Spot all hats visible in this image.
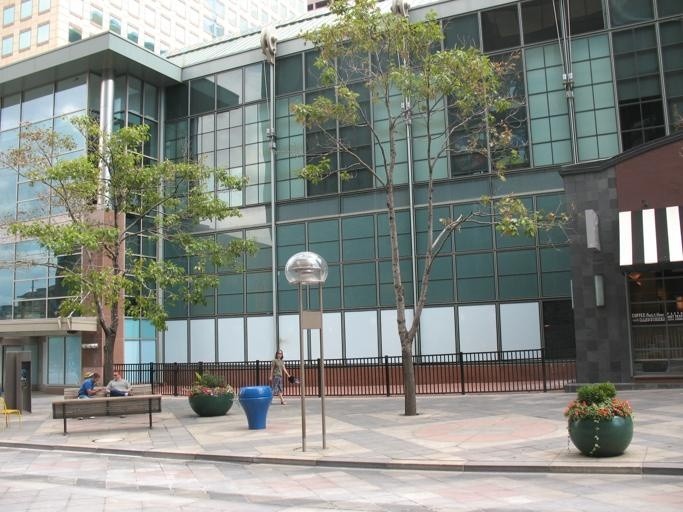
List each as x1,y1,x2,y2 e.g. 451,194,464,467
84,372,94,378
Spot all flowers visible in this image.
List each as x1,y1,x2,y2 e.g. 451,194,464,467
563,381,633,454
190,375,234,397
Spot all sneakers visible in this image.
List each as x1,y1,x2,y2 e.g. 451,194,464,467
128,391,135,396
281,400,288,406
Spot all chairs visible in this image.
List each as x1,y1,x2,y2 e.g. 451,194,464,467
0,398,20,428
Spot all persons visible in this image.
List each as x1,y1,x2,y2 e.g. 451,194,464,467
104,370,131,419
75,373,102,420
267,349,291,405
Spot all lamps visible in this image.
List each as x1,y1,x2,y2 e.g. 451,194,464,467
594,274,605,307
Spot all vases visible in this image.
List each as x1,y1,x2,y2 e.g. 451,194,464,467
643,360,668,373
569,414,632,457
189,396,234,417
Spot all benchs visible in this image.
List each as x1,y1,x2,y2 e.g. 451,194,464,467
64,384,152,400
53,394,162,433
